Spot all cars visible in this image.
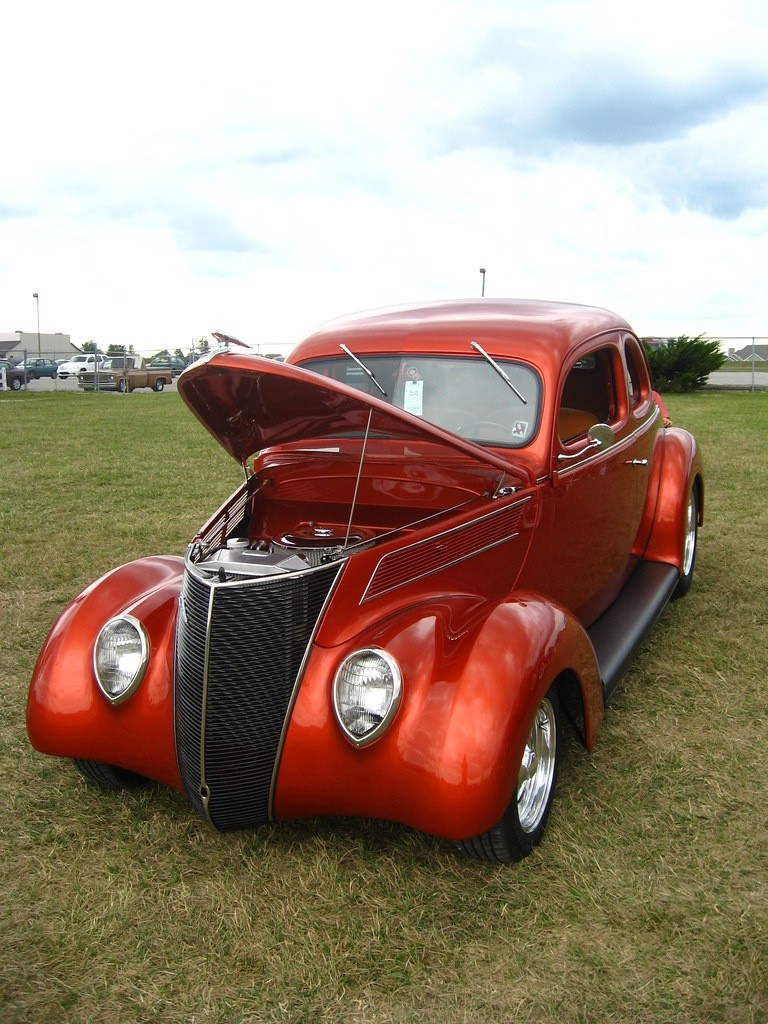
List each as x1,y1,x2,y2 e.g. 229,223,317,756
26,298,705,864
0,354,189,392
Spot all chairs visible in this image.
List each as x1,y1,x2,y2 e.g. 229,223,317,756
416,408,478,439
487,407,601,442
